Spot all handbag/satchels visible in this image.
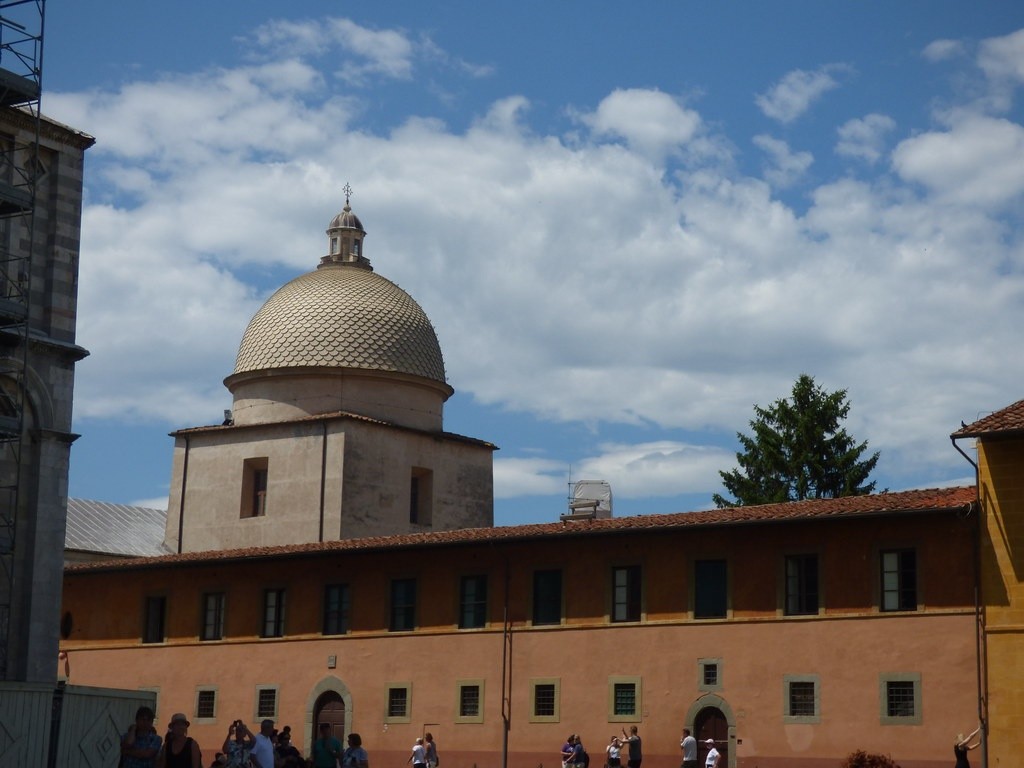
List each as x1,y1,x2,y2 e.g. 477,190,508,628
583,752,589,768
435,756,440,767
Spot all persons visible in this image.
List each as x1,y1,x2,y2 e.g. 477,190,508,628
160,713,203,768
560,734,585,768
953,725,981,768
222,719,255,768
424,732,438,768
120,706,162,768
408,737,428,768
211,753,225,768
338,733,368,768
606,726,642,768
704,739,721,768
311,723,344,768
251,719,307,768
679,728,697,768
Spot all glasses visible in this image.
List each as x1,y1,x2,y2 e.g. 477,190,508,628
282,737,290,741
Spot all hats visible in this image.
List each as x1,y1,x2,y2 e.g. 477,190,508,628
168,713,191,729
704,739,714,744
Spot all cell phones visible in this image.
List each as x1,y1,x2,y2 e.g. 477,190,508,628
233,721,238,727
